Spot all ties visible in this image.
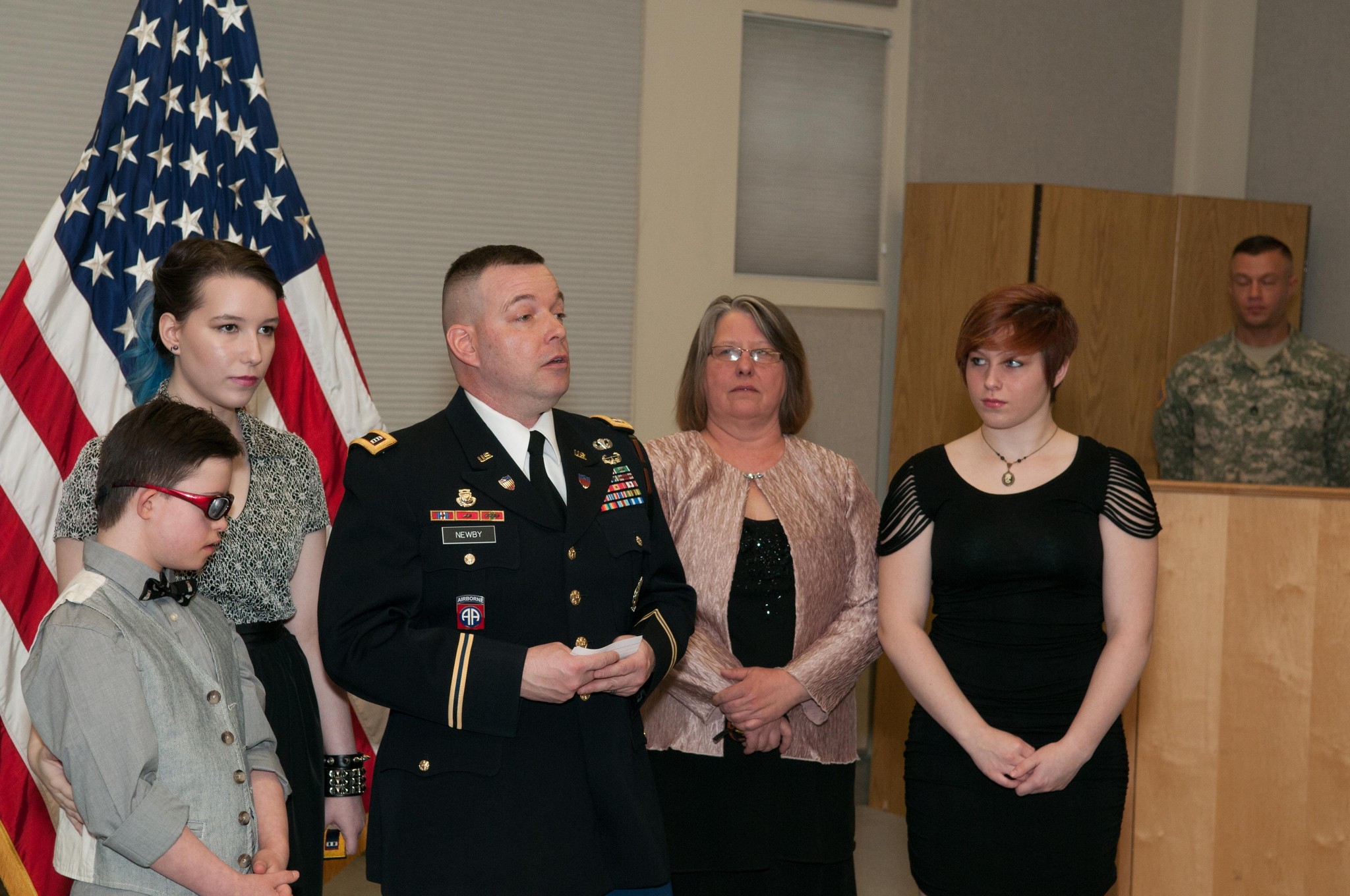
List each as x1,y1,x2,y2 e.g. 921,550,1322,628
527,428,567,520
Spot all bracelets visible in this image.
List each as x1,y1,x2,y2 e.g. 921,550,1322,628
324,752,371,798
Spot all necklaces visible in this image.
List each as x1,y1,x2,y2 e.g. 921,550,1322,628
981,425,1058,486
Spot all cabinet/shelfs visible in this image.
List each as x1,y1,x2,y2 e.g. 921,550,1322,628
869,180,1311,813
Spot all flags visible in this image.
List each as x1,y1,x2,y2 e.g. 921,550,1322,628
0,0,390,896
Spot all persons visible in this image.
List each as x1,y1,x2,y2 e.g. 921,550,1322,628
26,238,369,896
20,397,300,896
875,282,1162,896
1150,235,1350,489
318,245,699,896
627,295,884,896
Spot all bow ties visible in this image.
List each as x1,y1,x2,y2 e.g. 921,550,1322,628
138,572,197,607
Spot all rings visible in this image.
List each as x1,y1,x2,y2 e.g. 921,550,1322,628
611,690,617,694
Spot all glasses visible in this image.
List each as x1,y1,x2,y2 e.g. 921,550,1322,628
112,480,234,521
708,345,784,364
